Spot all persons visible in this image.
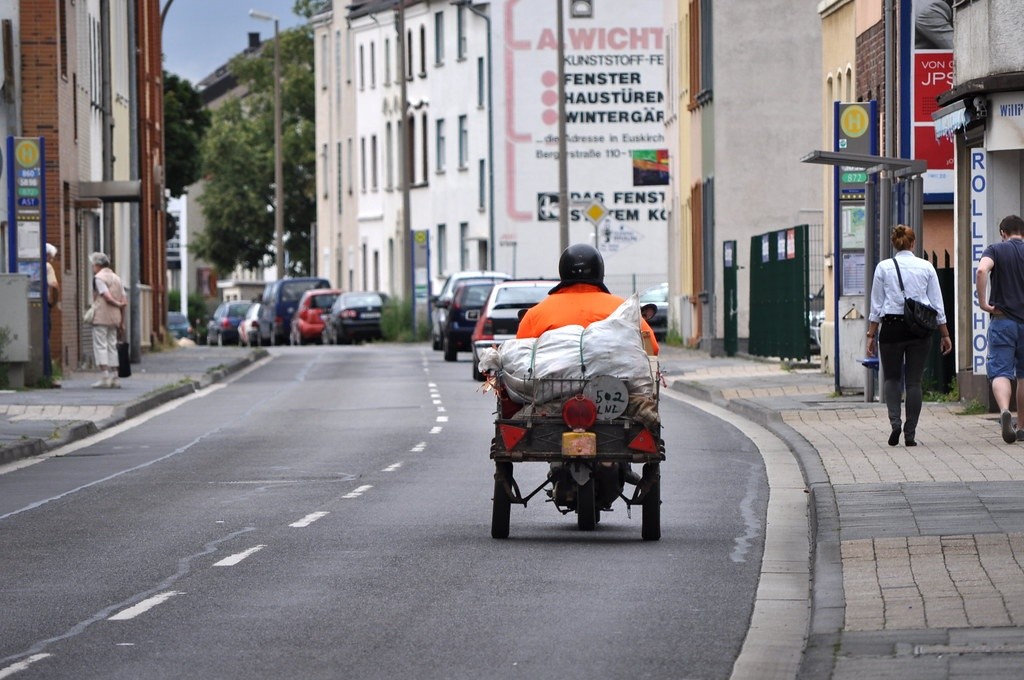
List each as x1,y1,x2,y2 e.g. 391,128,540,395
866,225,952,446
45,243,61,388
84,252,128,389
976,215,1024,444
516,243,660,501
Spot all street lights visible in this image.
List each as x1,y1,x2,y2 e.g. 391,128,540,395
249,9,284,282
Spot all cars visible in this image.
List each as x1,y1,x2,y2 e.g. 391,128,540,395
204,300,255,347
638,282,669,334
237,303,262,348
166,311,197,342
427,271,513,350
434,280,505,362
287,288,344,347
323,291,389,345
466,279,563,382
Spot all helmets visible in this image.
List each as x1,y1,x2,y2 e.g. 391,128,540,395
558,243,605,282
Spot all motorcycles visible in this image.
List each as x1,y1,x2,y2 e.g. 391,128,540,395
489,304,666,542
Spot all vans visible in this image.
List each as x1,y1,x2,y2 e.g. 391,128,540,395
255,275,331,347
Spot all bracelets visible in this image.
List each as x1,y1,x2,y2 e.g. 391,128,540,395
941,335,949,337
867,331,874,337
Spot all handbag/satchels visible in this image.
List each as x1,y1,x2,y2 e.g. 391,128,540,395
116,331,131,377
904,298,938,338
83,307,95,323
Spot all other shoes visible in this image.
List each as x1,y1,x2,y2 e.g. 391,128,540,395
626,463,640,485
1016,429,1024,441
91,381,112,388
112,381,121,388
52,380,61,388
905,441,918,446
888,425,902,446
998,409,1015,444
